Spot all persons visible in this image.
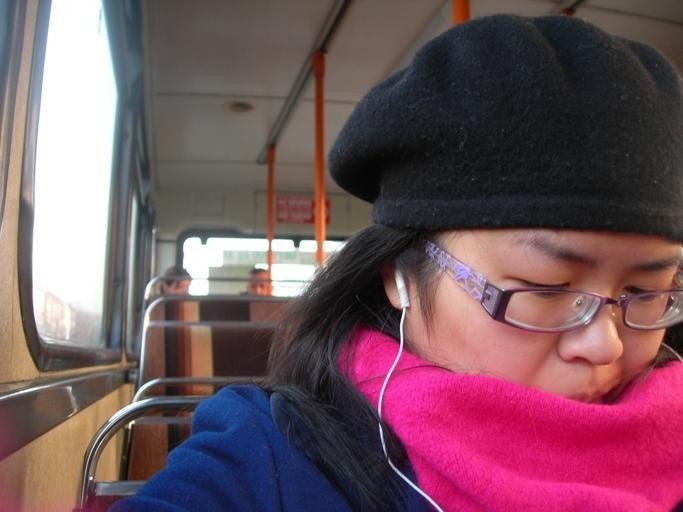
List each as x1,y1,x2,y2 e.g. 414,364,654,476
106,14,683,510
237,268,273,296
156,264,192,295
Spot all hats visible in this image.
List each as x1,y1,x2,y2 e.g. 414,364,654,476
331,13,682,236
163,266,192,280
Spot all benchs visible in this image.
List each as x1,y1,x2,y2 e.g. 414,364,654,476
78,277,302,511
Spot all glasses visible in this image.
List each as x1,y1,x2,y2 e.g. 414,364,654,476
417,240,683,332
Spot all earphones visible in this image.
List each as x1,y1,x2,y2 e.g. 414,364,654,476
391,261,410,309
